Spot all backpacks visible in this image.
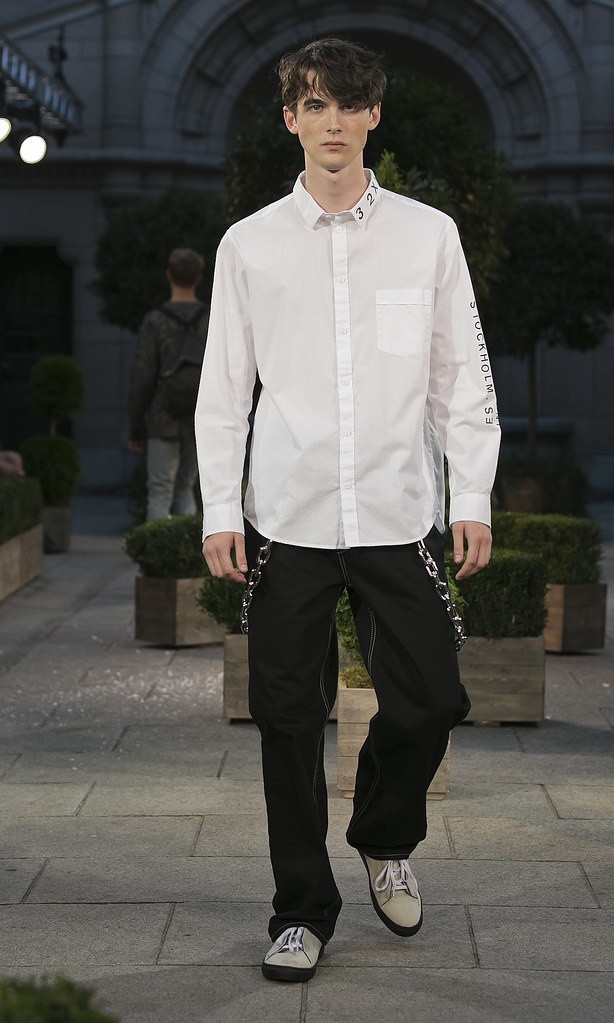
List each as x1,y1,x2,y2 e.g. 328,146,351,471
161,308,208,419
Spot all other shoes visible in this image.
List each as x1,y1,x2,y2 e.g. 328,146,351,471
261,923,326,981
357,851,424,938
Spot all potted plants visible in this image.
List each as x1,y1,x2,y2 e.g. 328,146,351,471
496,459,551,512
332,567,469,801
23,434,82,554
197,570,251,723
509,513,607,655
121,515,228,646
0,475,47,600
444,548,549,727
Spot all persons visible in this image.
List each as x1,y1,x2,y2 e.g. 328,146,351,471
191,35,505,983
126,250,213,525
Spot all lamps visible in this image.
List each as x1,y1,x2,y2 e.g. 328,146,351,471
11,127,48,163
